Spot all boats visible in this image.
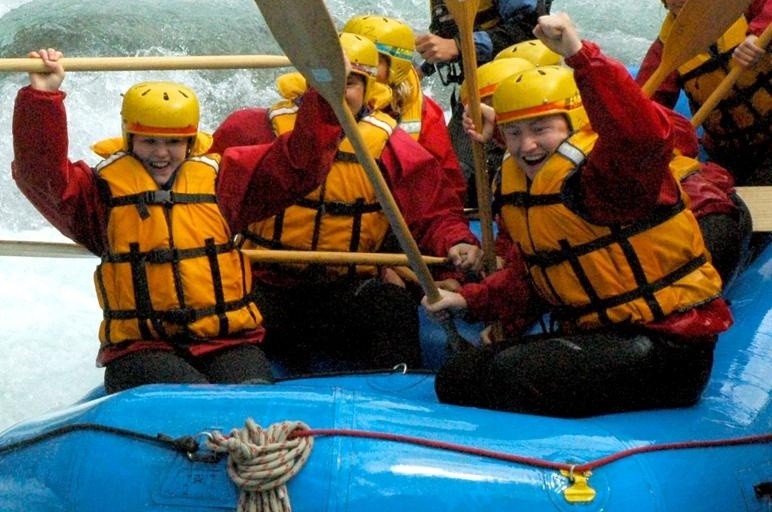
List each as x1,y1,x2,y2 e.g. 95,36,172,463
1,38,772,511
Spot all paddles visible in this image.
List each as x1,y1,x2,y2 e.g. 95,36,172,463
258,1,454,344
639,0,751,95
0,239,445,268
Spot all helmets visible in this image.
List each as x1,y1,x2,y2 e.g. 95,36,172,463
462,38,590,132
338,30,377,110
122,82,199,160
345,16,415,90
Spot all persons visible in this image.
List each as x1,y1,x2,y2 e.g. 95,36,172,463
210,1,752,415
10,47,350,394
634,1,772,184
